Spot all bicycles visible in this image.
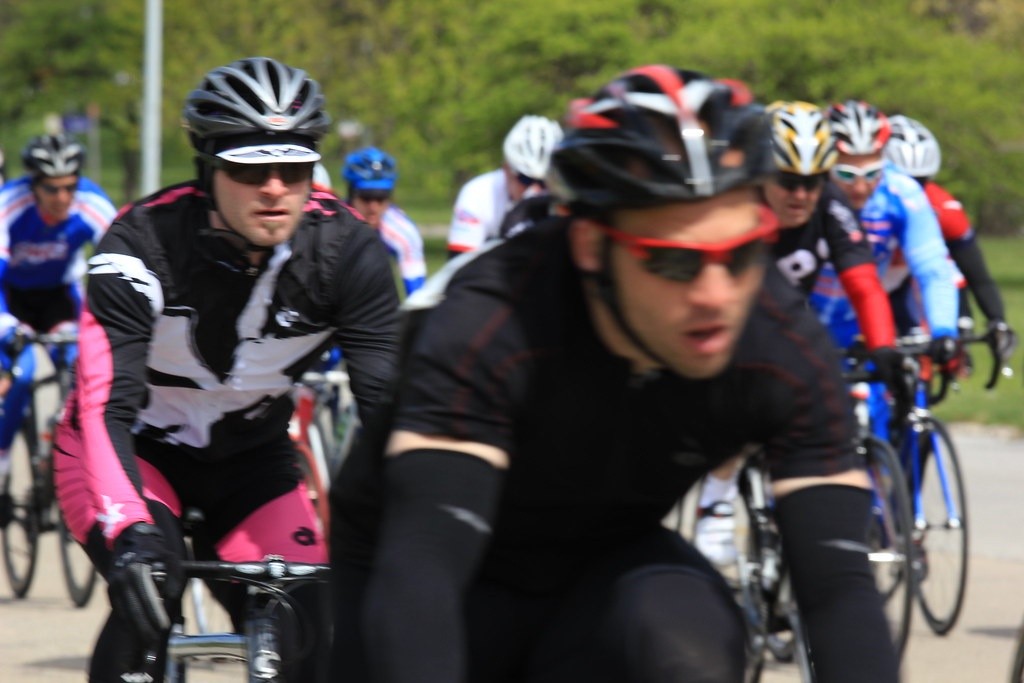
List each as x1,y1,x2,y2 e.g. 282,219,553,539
0,309,1013,683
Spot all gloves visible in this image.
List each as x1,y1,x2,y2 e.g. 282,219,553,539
928,336,956,363
869,347,910,419
108,521,180,637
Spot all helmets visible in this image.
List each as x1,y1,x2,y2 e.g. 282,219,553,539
823,100,892,154
544,66,774,207
879,115,940,176
23,133,84,177
503,115,563,179
342,148,396,189
183,57,332,164
764,101,840,174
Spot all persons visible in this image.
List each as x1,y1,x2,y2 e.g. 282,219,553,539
0,57,1014,683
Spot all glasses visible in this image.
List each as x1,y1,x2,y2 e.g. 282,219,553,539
588,208,777,282
40,182,78,193
772,170,818,191
830,164,882,182
218,163,314,185
358,189,389,200
515,172,545,190
